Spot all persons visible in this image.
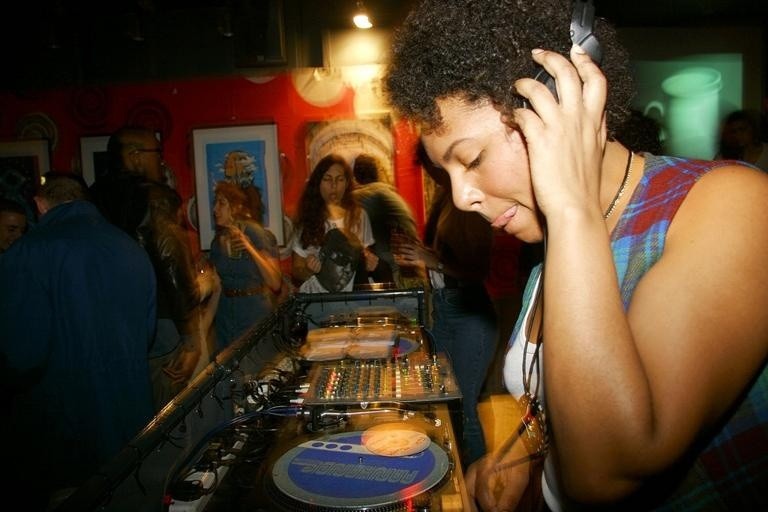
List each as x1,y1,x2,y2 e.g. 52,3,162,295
712,107,768,172
614,107,665,154
378,0,766,511
1,121,502,512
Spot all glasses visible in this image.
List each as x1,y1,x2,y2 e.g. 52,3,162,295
325,247,356,269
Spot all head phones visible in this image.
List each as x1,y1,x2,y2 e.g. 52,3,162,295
523,0,608,109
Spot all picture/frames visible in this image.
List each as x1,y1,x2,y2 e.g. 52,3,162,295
6,139,49,227
77,133,164,188
191,120,285,250
227,0,288,68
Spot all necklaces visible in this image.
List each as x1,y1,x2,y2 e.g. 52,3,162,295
514,150,636,463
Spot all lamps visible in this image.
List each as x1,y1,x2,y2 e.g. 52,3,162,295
352,0,377,29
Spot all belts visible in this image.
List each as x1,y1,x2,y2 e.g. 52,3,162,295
224,287,271,295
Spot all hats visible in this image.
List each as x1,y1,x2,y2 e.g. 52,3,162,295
322,230,365,270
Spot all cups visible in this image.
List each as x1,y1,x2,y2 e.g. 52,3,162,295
389,222,410,254
224,221,240,259
643,66,723,168
